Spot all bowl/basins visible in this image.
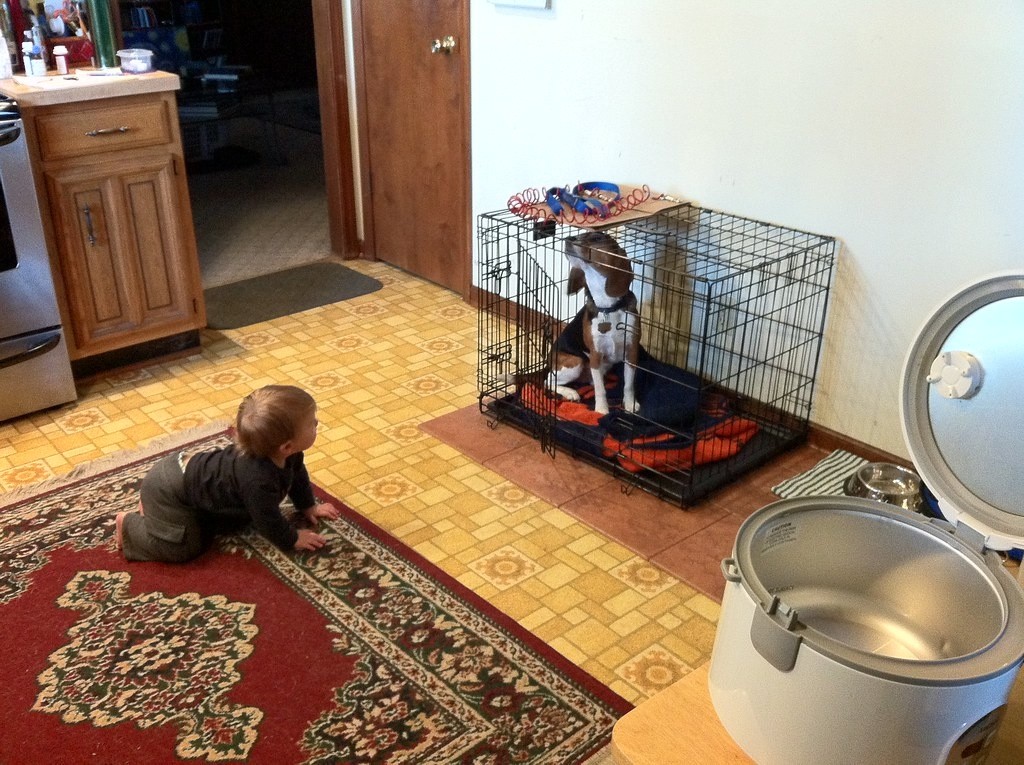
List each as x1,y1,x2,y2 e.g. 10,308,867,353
844,461,922,514
115,48,153,73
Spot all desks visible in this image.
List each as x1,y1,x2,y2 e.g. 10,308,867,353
611,555,1024,765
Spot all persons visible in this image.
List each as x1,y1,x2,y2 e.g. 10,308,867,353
115,385,341,562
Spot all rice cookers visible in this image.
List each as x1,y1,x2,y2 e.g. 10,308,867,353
707,260,1024,765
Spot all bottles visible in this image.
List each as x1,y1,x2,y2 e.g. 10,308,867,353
27,47,48,77
85,0,118,69
22,30,34,76
33,25,46,62
53,46,70,75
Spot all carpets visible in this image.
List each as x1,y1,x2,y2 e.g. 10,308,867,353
770,448,872,500
0,420,638,764
201,259,384,332
415,391,869,608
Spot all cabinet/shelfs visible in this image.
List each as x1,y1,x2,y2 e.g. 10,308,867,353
0,64,208,387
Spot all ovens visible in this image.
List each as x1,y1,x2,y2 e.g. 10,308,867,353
0,113,78,427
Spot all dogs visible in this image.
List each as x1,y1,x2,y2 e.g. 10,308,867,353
494,231,642,414
